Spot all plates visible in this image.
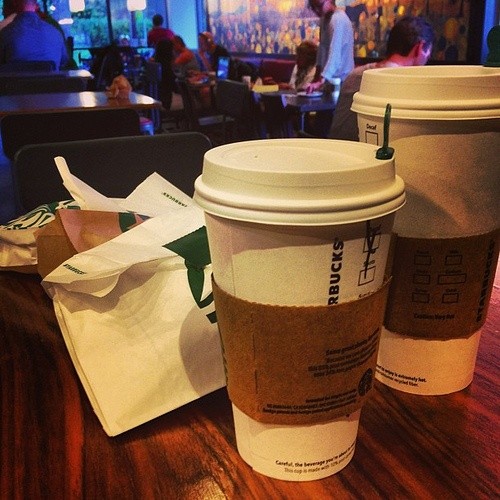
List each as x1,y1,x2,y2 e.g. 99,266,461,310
298,92,323,97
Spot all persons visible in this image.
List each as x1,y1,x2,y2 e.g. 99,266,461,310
114,13,234,134
264,41,317,91
0,0,69,71
328,16,435,141
306,0,354,128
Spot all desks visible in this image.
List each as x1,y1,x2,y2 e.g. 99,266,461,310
0,250,500,500
0,69,93,91
0,92,163,157
259,89,339,131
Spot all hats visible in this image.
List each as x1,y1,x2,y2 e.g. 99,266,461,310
199,30,214,43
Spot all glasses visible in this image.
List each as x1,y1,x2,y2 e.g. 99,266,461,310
307,0,326,11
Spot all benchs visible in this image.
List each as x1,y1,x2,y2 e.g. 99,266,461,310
230,56,296,82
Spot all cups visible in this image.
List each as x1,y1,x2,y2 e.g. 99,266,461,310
350,64,500,398
192,138,408,482
242,75,251,90
329,77,341,96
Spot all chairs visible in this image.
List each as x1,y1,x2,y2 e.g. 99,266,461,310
13,131,212,221
0,61,69,90
96,54,293,141
1,109,140,159
